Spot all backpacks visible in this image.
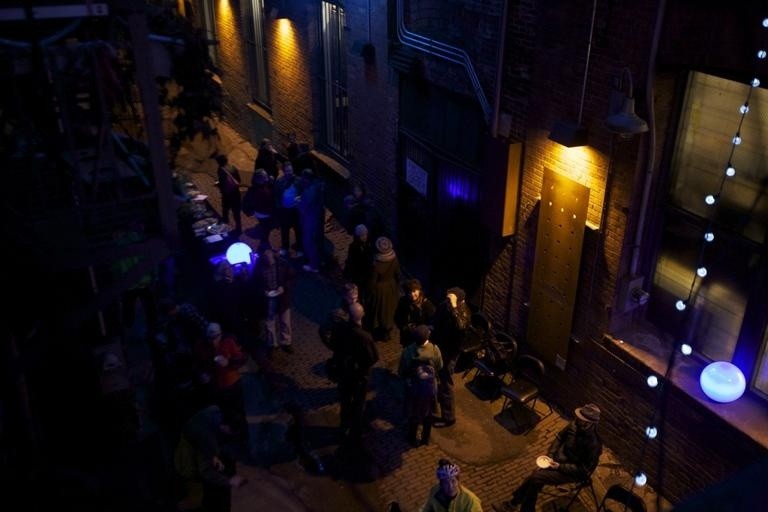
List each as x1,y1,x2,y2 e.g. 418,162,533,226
414,366,437,398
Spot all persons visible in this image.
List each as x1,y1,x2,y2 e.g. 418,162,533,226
490,403,603,512
0,3,346,512
345,183,470,448
419,458,482,511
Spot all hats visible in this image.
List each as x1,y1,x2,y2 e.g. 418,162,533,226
375,236,393,255
575,404,601,424
205,322,223,340
353,224,368,237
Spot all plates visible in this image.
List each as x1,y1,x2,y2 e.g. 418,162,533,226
535,455,552,470
268,290,280,298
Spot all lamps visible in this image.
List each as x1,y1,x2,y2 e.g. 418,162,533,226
548,116,593,148
270,6,287,19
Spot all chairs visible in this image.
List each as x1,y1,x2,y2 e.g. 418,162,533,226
454,313,553,435
557,459,647,511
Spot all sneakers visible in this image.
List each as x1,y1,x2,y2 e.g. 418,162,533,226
433,418,455,428
268,343,296,359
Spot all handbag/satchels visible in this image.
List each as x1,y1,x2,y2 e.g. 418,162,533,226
217,165,240,193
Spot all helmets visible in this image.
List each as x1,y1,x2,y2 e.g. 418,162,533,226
436,463,461,480
